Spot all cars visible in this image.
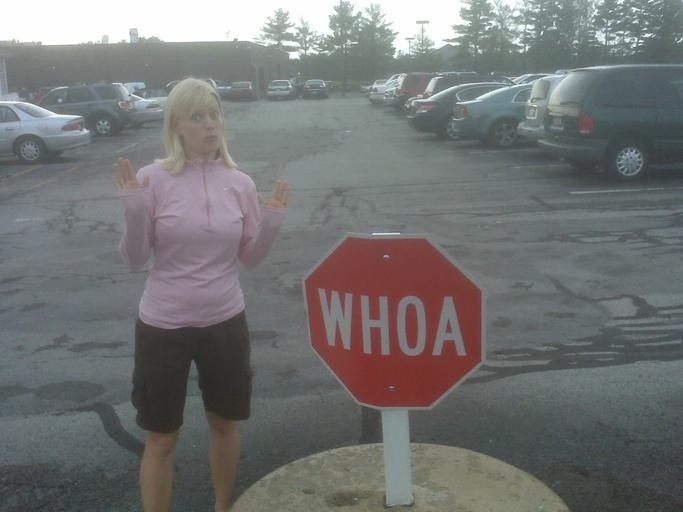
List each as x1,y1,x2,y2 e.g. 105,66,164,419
0,100,92,164
129,93,162,129
360,63,567,150
131,87,168,119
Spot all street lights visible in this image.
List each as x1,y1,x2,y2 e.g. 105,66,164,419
405,37,415,55
416,20,430,51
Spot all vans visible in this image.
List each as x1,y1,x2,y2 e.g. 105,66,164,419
536,63,682,181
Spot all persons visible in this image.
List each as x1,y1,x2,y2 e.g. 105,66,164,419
114,73,291,511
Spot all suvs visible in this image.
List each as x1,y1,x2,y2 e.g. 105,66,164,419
206,77,332,101
34,82,134,140
